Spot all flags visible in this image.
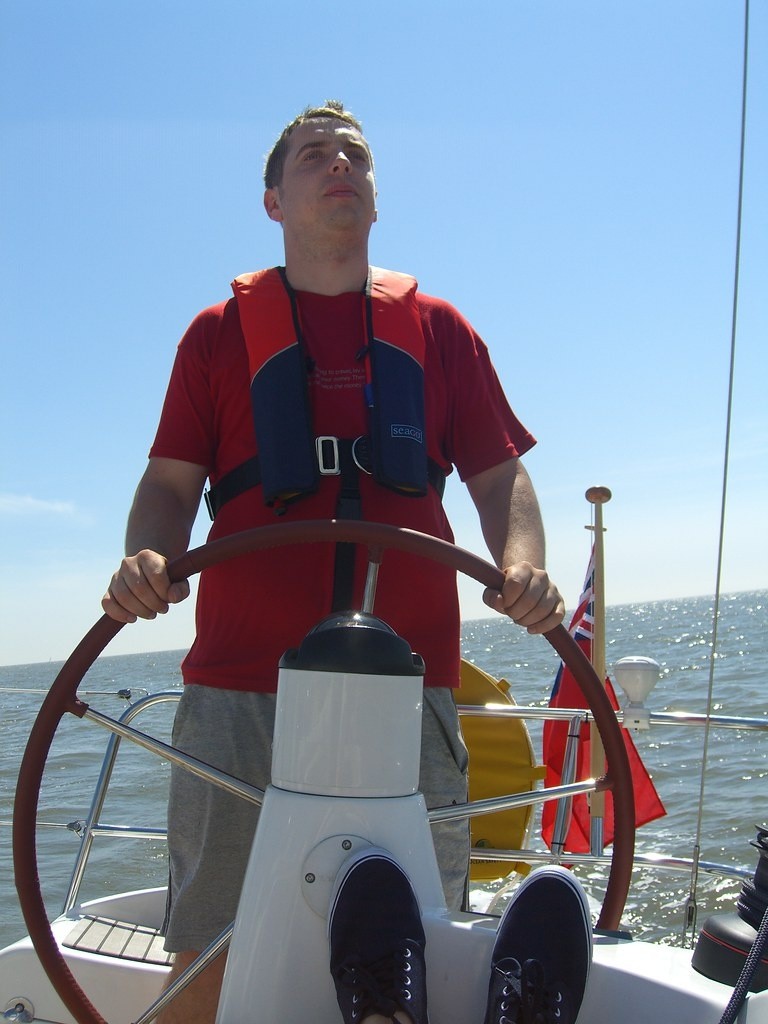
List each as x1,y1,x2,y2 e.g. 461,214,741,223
542,542,669,871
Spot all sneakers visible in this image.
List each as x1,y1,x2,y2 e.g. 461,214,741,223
328,845,431,1024
483,864,593,1024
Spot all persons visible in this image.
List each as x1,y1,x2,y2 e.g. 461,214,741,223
327,847,593,1024
100,103,565,1024
